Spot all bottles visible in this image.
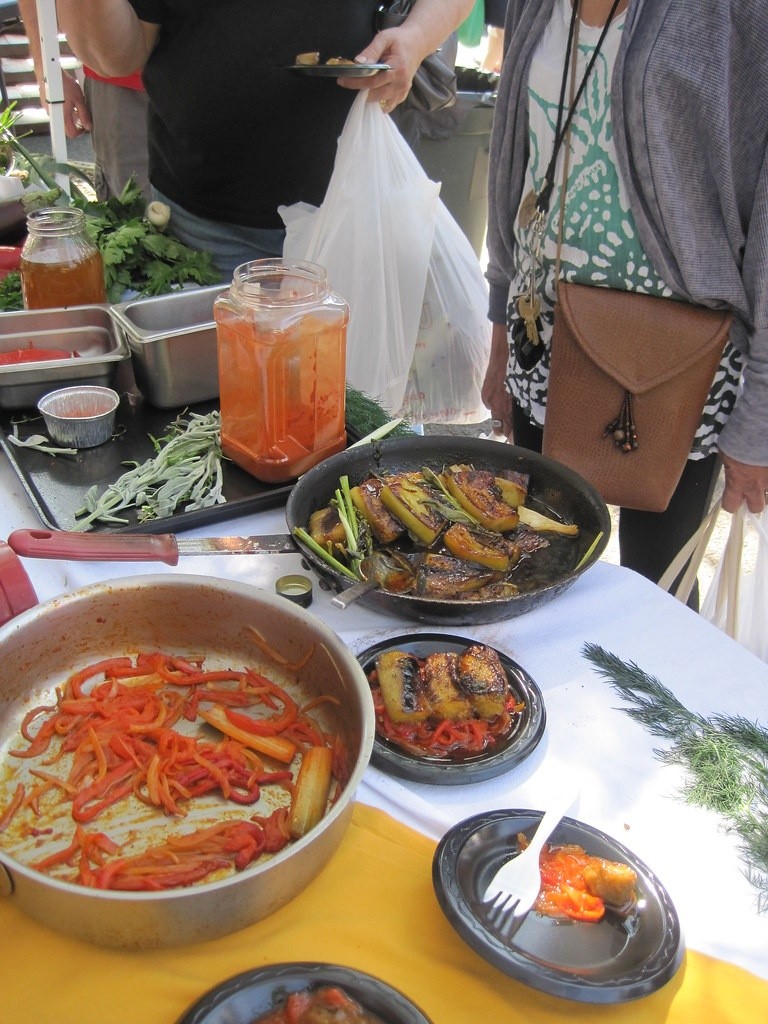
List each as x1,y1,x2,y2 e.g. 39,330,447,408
19,205,105,310
212,256,350,484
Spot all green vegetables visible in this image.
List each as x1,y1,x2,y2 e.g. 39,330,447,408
0,99,228,312
6,375,417,531
297,467,480,582
581,641,768,904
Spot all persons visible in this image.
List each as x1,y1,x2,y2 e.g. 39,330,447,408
481,0,768,613
18,0,153,222
56,0,474,298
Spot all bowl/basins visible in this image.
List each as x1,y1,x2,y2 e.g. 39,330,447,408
36,384,120,449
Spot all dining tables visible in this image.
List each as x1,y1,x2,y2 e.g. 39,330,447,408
0,283,768,1024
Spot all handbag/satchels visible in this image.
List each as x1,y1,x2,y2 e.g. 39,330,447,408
369,0,459,114
541,279,732,514
276,84,493,426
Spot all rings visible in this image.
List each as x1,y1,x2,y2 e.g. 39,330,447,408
381,99,387,109
765,492,768,494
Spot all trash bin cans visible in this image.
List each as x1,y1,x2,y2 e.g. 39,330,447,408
409,64,501,265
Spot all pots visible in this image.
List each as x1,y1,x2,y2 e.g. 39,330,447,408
0,572,376,953
6,435,612,629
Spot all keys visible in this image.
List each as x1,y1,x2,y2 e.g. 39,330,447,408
516,296,540,345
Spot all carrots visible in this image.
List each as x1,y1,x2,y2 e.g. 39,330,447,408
0,652,353,891
367,671,521,755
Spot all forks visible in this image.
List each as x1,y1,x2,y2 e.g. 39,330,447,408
481,783,576,919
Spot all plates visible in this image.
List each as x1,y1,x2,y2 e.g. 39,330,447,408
273,63,392,78
432,808,684,1005
356,633,548,786
175,962,432,1023
